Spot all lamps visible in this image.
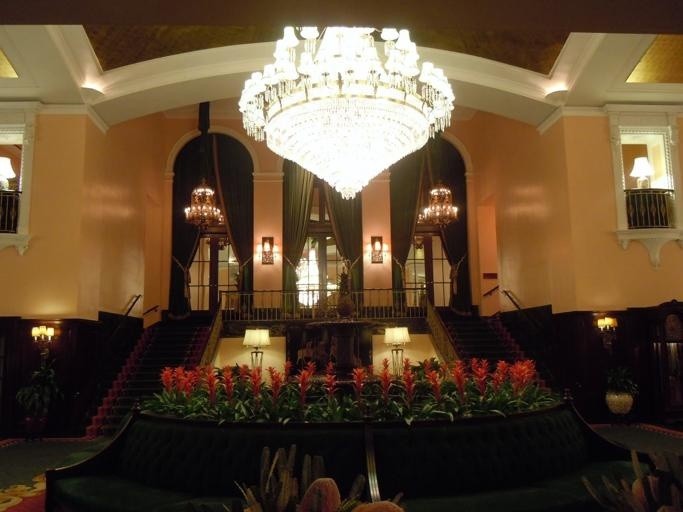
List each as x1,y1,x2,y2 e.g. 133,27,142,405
237,22,456,205
416,178,460,227
0,155,17,190
596,315,620,349
629,157,654,189
243,327,270,365
29,324,56,366
185,177,225,232
383,327,412,366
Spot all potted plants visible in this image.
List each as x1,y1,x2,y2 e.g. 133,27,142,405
14,359,66,442
602,366,640,415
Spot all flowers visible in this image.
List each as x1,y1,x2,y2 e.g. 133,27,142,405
147,358,553,422
